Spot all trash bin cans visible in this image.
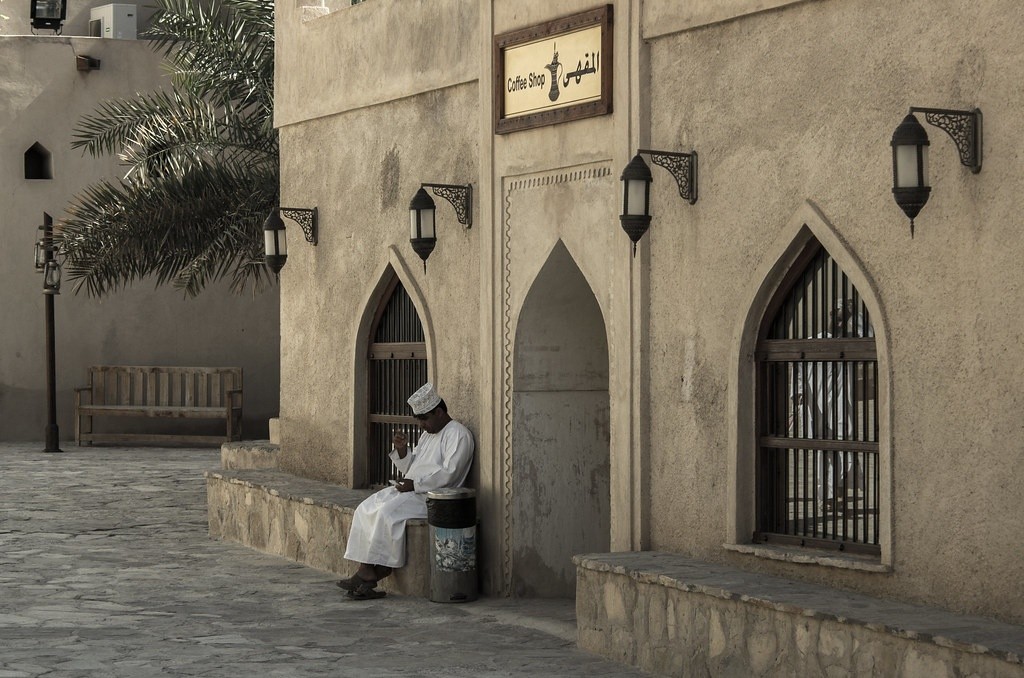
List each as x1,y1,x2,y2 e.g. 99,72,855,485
427,487,479,602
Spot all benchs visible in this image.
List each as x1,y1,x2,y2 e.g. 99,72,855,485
72,365,246,452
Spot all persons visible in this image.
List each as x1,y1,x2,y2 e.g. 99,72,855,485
791,298,862,511
336,383,474,599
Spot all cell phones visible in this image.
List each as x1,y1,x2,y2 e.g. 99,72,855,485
389,480,401,486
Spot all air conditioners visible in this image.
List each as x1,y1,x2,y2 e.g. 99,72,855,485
88,3,138,40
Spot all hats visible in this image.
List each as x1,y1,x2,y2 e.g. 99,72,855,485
407,383,441,415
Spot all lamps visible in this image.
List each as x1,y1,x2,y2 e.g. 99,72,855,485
620,149,698,257
889,107,984,241
263,206,319,286
29,0,67,35
407,182,474,274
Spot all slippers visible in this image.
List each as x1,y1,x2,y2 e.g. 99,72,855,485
345,584,386,599
336,573,377,590
